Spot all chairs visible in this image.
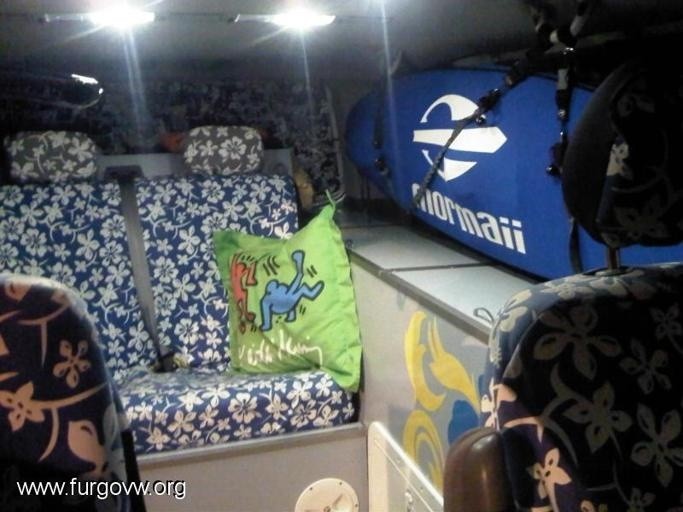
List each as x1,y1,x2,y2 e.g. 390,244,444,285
0,75,365,511
448,46,683,512
